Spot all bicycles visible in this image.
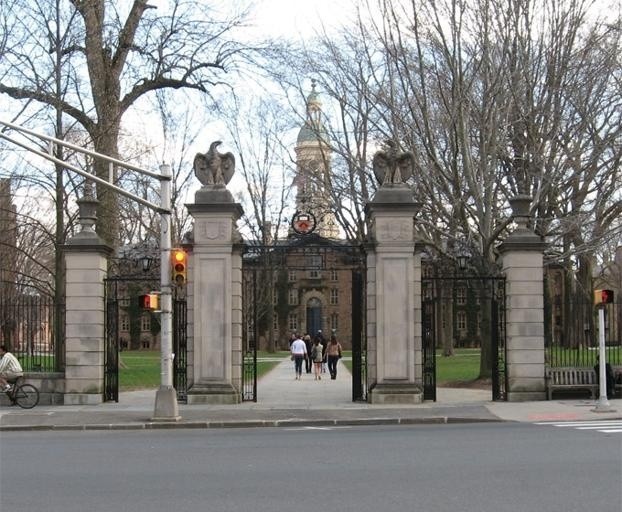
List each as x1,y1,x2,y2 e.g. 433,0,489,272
0,375,41,409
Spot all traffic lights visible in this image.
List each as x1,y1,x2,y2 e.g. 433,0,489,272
593,288,616,307
172,250,187,285
144,293,158,310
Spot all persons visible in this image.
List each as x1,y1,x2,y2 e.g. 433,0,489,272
594,355,616,396
288,328,343,380
0,345,24,394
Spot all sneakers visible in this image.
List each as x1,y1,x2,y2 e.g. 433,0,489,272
1,384,11,392
295,368,337,380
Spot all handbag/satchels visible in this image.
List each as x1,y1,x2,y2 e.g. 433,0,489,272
338,351,342,358
311,349,317,359
